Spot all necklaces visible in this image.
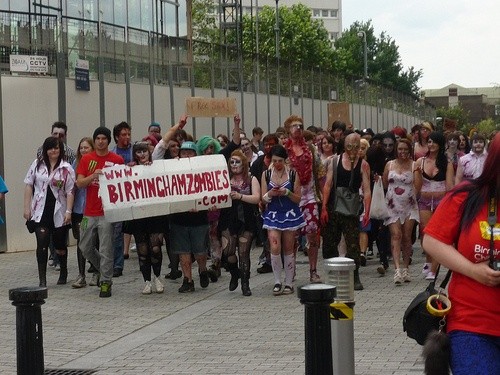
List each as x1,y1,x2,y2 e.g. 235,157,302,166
280,169,285,177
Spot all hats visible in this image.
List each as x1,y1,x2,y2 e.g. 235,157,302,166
361,128,374,137
93,126,111,145
180,141,196,152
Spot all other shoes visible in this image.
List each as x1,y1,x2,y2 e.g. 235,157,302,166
360,254,366,266
39,255,323,297
402,268,411,282
354,275,363,290
377,265,385,274
422,262,432,274
425,272,436,280
366,251,373,258
393,268,401,284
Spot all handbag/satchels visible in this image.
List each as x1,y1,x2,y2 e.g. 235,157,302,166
333,187,360,216
360,176,391,221
26,218,35,233
403,290,446,345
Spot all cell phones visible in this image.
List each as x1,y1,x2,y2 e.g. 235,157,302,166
273,186,280,189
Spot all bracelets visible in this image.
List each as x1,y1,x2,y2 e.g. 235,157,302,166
284,190,290,195
239,195,242,200
66,209,72,214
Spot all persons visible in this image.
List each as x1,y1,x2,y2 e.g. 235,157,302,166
422,131,500,375
0,177,8,203
24,117,487,296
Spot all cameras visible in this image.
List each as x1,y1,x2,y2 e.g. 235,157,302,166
489,259,500,271
